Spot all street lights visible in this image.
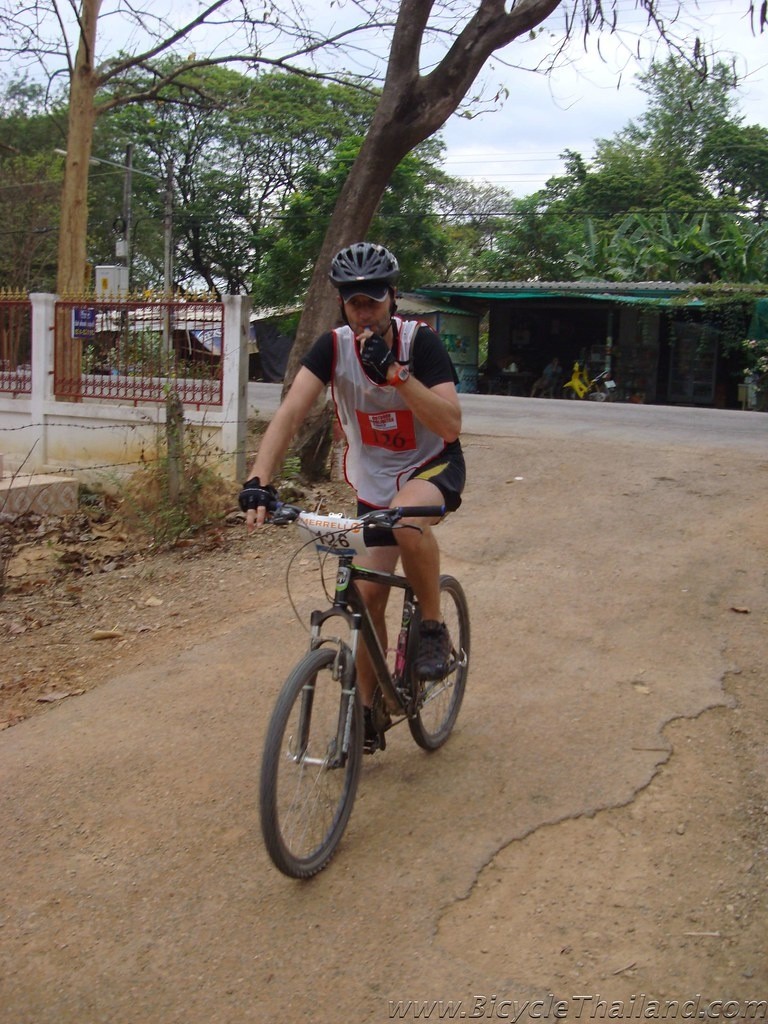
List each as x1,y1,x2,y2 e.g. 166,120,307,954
53,148,171,376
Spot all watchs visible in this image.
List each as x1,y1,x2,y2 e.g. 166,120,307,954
389,366,409,386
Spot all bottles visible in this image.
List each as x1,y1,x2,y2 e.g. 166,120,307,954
360,325,374,354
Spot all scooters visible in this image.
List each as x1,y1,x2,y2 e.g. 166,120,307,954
562,359,621,403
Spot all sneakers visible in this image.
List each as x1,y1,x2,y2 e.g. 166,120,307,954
413,620,449,680
363,705,392,747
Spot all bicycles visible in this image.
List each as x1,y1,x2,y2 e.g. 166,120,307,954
232,502,470,879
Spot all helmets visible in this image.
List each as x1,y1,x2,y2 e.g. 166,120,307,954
328,242,400,288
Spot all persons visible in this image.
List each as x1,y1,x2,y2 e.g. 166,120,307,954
529,356,563,397
237,241,467,751
